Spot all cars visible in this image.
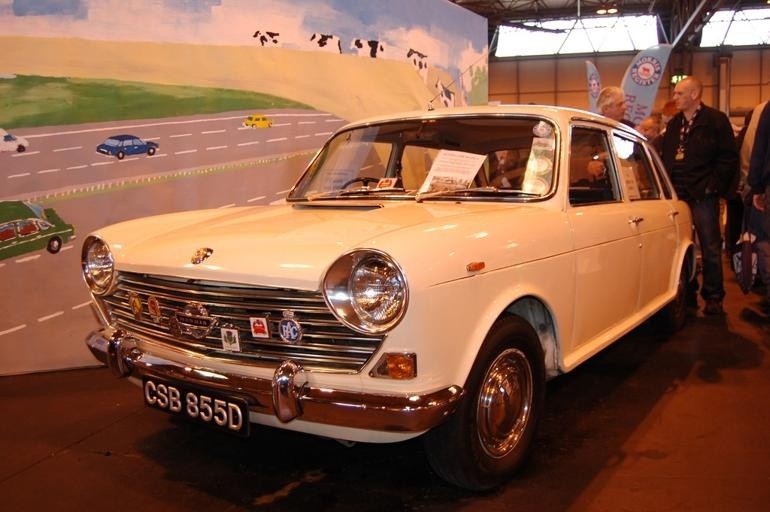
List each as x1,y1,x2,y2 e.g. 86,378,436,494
81,101,696,490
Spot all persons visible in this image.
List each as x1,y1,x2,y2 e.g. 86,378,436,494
725,100,770,315
662,75,737,316
595,86,637,129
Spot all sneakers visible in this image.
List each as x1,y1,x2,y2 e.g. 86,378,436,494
703,297,724,314
